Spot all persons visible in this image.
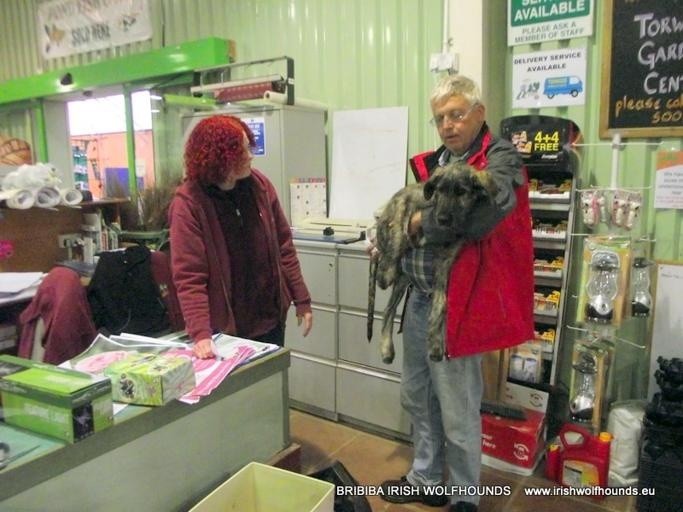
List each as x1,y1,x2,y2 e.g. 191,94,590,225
367,74,535,512
169,113,313,359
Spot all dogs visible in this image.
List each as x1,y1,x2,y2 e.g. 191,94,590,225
367,162,499,365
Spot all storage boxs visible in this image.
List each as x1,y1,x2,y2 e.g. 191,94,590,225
479,406,546,476
0,354,114,445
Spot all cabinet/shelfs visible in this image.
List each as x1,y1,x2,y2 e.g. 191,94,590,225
507,177,579,396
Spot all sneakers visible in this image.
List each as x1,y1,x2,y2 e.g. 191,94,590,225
449,501,478,512
379,475,448,508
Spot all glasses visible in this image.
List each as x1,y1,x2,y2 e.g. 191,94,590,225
428,105,474,127
